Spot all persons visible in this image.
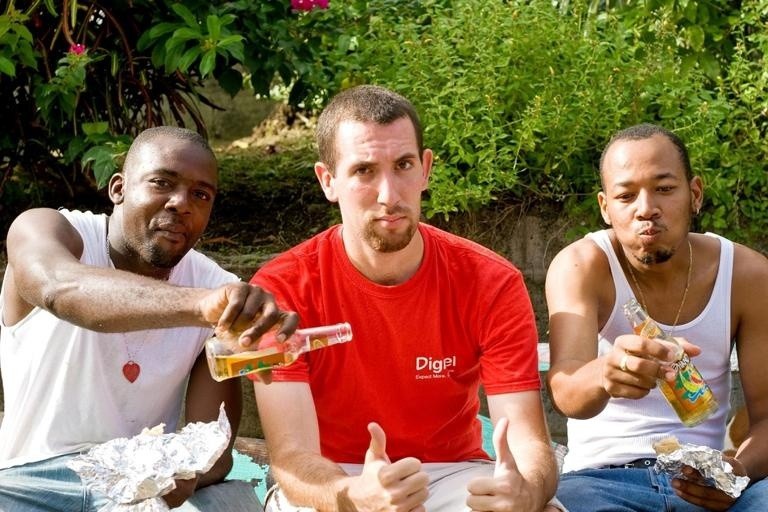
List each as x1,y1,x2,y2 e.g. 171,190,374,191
545,124,767,511
246,84,567,512
1,124,298,512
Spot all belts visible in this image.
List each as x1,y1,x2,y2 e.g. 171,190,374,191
605,458,656,469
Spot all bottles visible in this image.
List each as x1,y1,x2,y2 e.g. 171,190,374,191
206,322,353,381
620,297,719,428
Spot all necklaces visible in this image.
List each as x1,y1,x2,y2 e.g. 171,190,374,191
623,240,692,338
118,330,154,384
